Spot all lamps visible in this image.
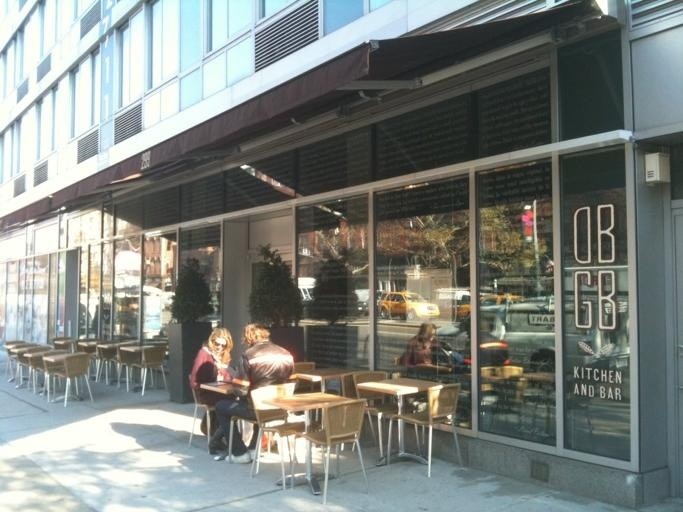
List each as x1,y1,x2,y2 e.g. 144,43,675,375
233,103,348,156
406,28,557,92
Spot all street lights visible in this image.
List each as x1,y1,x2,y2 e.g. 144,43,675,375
523,204,543,295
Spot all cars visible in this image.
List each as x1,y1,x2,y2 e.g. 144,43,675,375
353,288,372,318
375,283,629,410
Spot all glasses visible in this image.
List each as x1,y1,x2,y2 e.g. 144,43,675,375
214,341,226,347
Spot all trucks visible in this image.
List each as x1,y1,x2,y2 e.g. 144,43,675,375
296,277,318,302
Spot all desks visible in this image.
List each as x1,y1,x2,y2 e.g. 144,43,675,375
200,380,251,460
291,367,362,392
359,378,440,467
262,392,354,495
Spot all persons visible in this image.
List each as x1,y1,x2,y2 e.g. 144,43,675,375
191,326,236,461
216,322,295,465
399,320,441,365
449,313,504,370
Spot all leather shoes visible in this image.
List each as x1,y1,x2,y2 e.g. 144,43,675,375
216,441,226,450
210,446,216,454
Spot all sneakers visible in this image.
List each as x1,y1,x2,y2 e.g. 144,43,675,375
225,452,251,464
248,449,256,460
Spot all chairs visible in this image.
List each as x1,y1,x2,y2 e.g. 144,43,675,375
341,371,375,452
470,356,598,444
188,371,243,454
228,383,296,477
250,386,327,490
387,383,464,477
290,398,370,504
0,334,170,406
349,372,422,463
290,361,316,391
